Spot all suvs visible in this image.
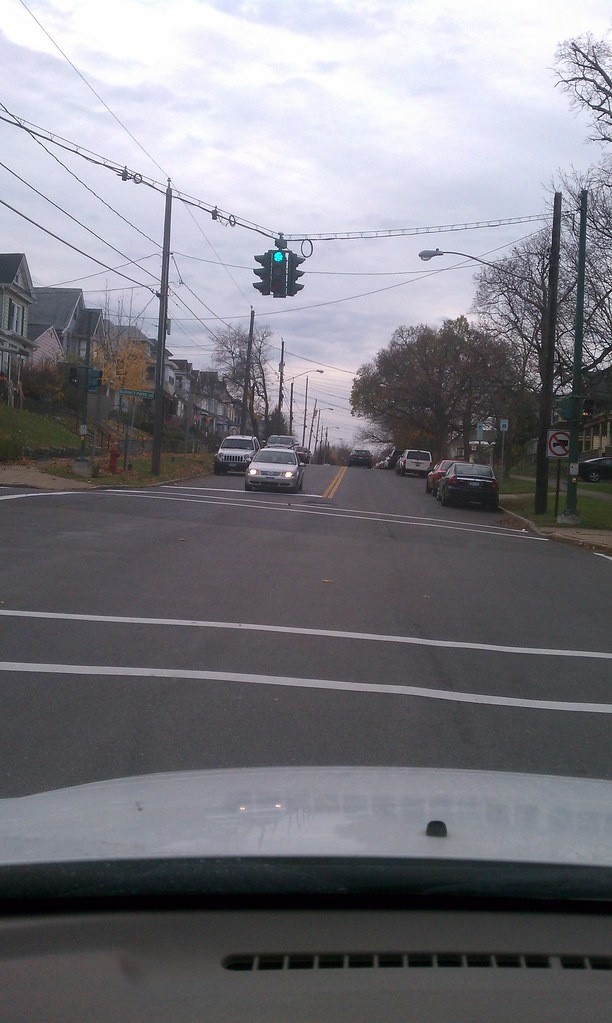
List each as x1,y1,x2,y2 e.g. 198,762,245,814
213,434,261,476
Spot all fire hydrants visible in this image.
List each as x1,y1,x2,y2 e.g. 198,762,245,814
108,443,121,471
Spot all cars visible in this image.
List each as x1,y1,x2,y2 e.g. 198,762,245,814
375,449,433,477
348,449,373,469
426,459,499,511
579,456,612,483
244,434,312,492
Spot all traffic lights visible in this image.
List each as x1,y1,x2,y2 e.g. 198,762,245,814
287,251,306,296
581,398,594,420
87,367,103,387
271,250,286,293
65,363,86,387
252,250,271,295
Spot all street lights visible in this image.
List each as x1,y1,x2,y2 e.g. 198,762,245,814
320,425,339,450
277,337,324,432
308,398,334,450
328,437,343,450
418,248,558,516
